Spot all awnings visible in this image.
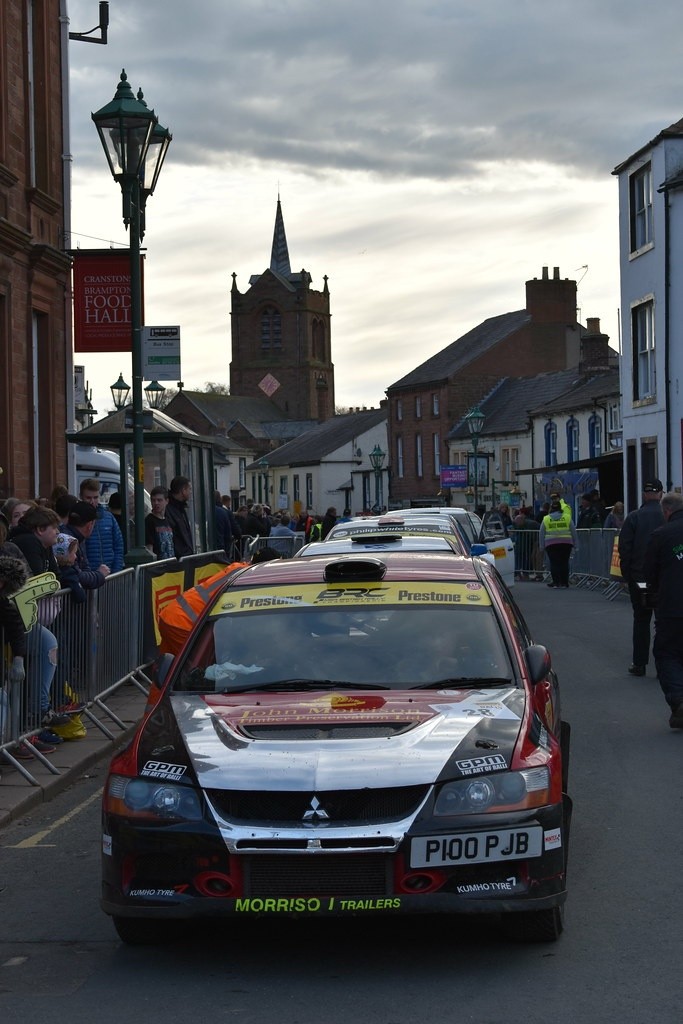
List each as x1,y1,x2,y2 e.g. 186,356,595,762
541,472,598,493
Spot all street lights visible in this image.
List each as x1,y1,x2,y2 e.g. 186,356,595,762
368,443,386,518
259,457,270,506
89,65,173,576
466,405,487,517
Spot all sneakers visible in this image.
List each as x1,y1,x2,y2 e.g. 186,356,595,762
48,697,87,714
9,743,34,758
33,706,70,727
38,731,62,744
27,736,56,753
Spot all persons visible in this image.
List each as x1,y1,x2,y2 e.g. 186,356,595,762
645,491,683,728
215,491,351,564
145,486,174,561
617,477,665,677
488,503,512,530
146,547,281,716
578,489,609,528
164,477,193,561
540,500,579,589
550,492,572,518
0,480,123,765
511,501,549,582
604,501,624,528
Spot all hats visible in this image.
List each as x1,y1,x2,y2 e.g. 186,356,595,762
550,493,560,498
68,502,97,521
644,478,662,492
52,533,76,557
253,546,286,561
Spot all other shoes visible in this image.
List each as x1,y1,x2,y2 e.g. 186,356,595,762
628,664,645,675
669,703,683,728
561,584,567,588
546,583,557,589
532,576,543,581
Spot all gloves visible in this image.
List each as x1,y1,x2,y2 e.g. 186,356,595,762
10,658,25,681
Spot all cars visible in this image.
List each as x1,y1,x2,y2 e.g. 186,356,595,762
100,505,574,946
